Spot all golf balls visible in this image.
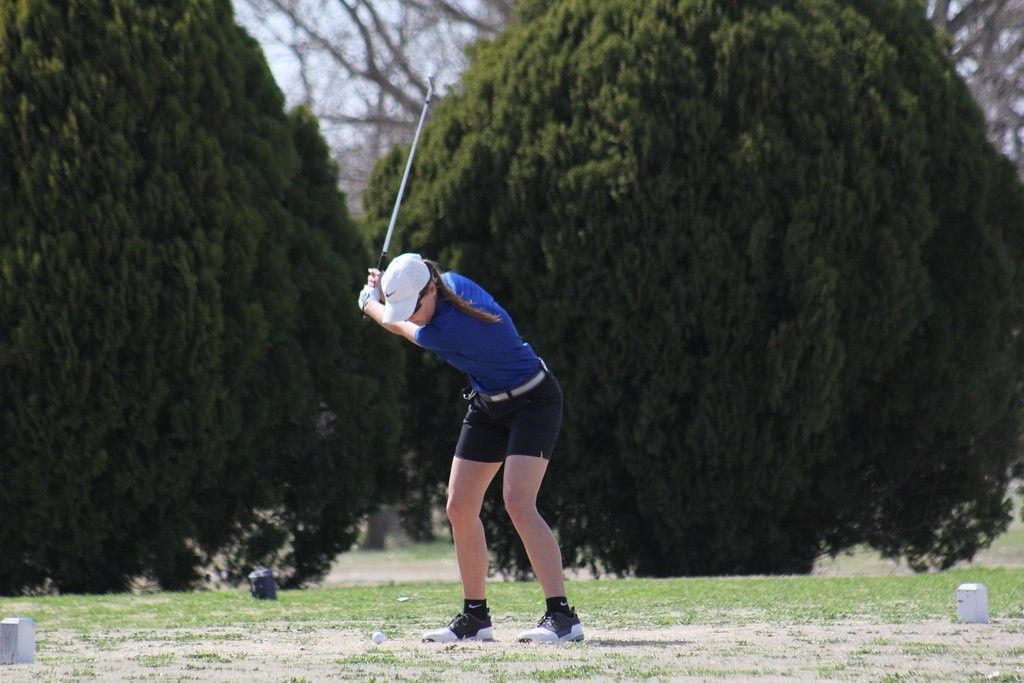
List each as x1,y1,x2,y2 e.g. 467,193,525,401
372,631,385,644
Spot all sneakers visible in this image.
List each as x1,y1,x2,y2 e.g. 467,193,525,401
518,606,584,644
422,611,493,643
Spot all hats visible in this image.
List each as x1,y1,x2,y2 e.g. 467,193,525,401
382,253,431,323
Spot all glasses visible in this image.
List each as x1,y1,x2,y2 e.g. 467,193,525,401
413,292,426,314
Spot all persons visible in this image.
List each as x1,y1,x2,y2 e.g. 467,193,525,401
358,252,586,645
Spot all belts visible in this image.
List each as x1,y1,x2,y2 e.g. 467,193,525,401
471,358,548,404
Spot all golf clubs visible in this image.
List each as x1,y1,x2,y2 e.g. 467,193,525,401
362,86,433,323
